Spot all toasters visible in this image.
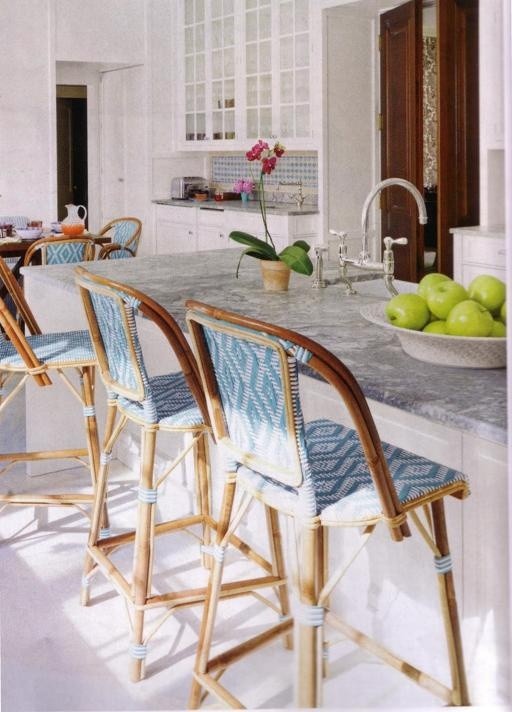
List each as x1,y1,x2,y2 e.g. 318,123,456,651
170,176,205,199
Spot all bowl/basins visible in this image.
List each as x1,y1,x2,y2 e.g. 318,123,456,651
192,190,209,200
16,228,43,240
51,223,64,233
0,229,16,238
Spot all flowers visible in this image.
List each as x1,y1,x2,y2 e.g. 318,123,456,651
234,179,254,195
228,139,314,280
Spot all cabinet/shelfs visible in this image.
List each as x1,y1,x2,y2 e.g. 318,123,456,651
177,0,321,151
454,234,507,289
156,204,197,253
198,210,257,251
257,215,318,251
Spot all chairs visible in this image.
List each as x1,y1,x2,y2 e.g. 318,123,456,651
1,216,42,316
75,265,292,682
1,255,110,539
16,236,109,336
183,299,472,711
99,218,142,260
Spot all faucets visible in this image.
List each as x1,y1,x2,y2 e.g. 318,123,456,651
360,177,429,258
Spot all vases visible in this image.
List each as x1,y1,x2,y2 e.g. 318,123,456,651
260,260,291,291
241,192,249,202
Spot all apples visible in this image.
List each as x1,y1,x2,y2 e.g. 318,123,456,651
445,299,494,337
488,319,507,337
421,321,445,335
500,300,506,324
426,281,467,320
467,274,506,318
418,273,453,323
386,293,429,330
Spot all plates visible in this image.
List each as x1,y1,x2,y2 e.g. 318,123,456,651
359,298,509,371
187,197,211,202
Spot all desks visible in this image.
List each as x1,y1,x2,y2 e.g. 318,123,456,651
0,235,111,299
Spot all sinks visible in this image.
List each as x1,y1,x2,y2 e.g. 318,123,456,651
325,271,422,300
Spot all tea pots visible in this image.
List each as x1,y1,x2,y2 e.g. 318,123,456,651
60,204,88,236
